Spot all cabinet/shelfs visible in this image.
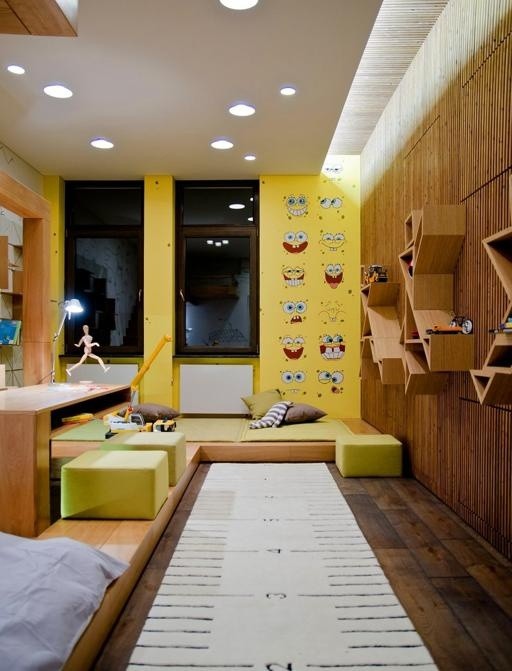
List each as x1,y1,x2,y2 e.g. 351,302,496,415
359,207,512,407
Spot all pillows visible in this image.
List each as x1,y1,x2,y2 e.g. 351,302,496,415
283,402,326,424
240,388,283,420
129,403,179,423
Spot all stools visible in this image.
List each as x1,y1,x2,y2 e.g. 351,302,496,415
335,434,404,479
101,429,187,485
61,450,169,520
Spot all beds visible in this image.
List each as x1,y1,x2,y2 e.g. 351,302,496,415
49,417,383,463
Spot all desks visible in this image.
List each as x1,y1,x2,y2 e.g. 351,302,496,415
0,382,130,538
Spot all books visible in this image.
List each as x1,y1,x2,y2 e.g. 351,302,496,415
0,318,23,346
487,315,512,333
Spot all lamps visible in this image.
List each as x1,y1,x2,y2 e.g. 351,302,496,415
35,298,84,385
